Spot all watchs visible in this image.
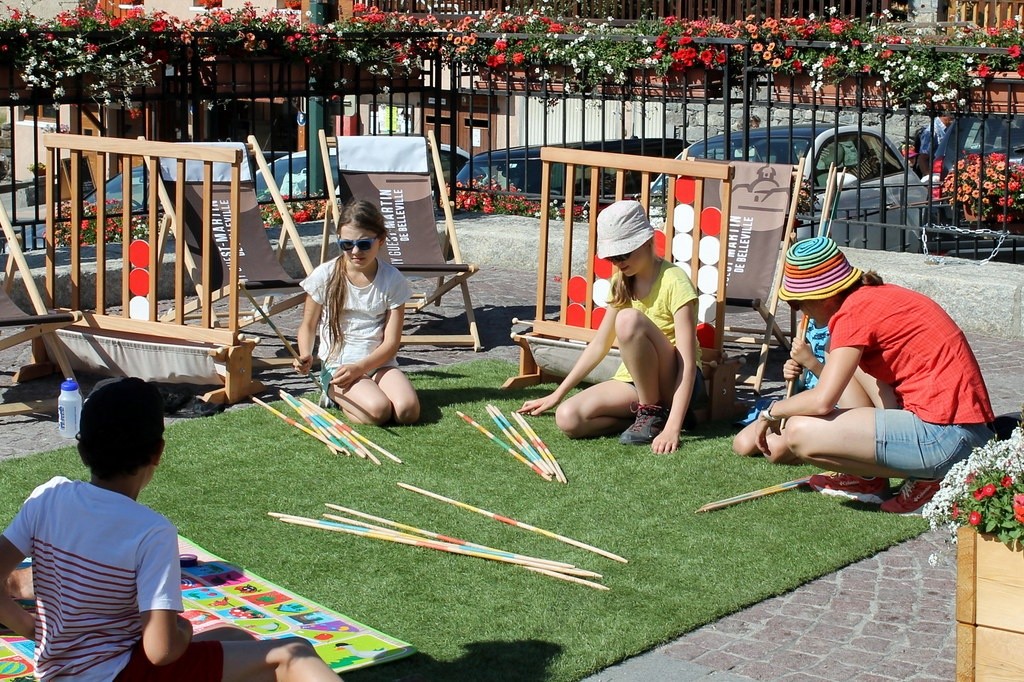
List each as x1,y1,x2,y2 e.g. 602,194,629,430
760,399,778,421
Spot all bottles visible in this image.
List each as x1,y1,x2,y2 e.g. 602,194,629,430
57,376,83,439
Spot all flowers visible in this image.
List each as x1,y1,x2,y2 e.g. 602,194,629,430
925,423,1024,550
0,0,1024,114
943,151,1024,224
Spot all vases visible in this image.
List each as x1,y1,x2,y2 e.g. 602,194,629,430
201,53,318,94
963,201,988,221
924,70,1024,113
332,57,421,90
955,528,1024,682
0,51,163,101
772,69,893,106
593,65,724,99
478,64,577,92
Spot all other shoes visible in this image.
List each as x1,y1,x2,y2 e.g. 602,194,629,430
319,389,337,409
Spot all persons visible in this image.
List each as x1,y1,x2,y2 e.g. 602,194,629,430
0,379,347,682
518,201,707,458
292,201,421,426
731,114,761,163
733,236,995,519
899,100,960,180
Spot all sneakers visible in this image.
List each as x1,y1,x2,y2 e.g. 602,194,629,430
879,478,940,516
810,471,893,504
618,400,669,445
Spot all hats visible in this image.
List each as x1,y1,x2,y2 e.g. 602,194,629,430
596,199,656,260
777,234,862,301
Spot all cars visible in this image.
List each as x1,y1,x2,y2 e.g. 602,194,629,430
81,150,292,223
255,147,492,223
637,125,931,255
923,115,1024,263
456,138,690,200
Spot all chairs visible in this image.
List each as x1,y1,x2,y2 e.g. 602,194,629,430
814,143,857,188
135,136,804,369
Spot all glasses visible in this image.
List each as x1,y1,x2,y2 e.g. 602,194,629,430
603,251,632,262
336,233,380,251
786,300,800,312
